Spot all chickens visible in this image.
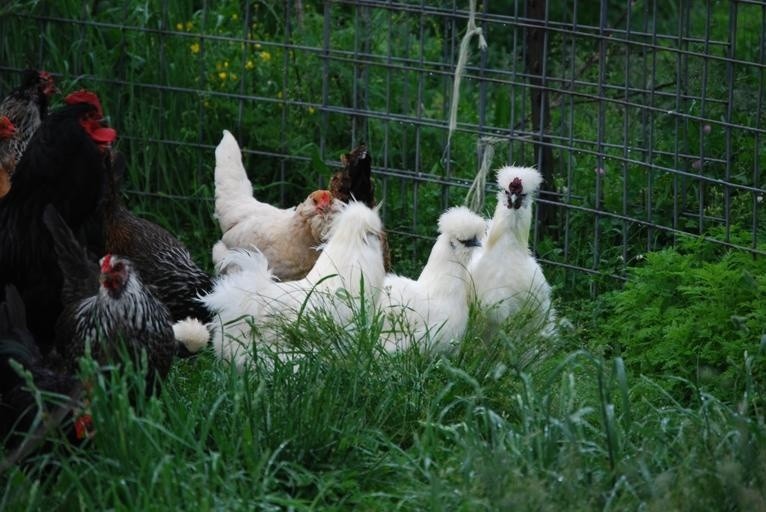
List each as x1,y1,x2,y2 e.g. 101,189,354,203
190,129,573,378
0,67,218,499
